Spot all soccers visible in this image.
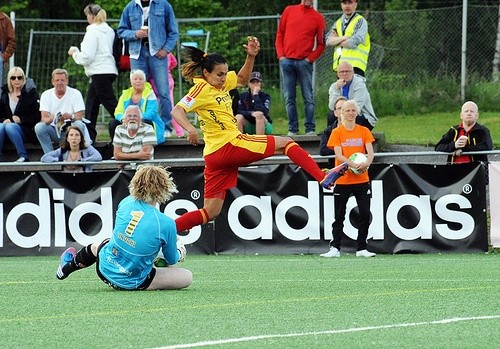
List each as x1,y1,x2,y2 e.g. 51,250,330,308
346,152,369,174
153,235,180,267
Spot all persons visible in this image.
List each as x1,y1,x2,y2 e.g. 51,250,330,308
56,165,192,292
320,98,348,169
329,61,377,130
0,67,41,162
148,52,184,138
112,105,158,160
34,69,86,153
41,126,103,172
117,0,178,137
173,36,348,234
434,101,493,186
320,100,376,259
275,0,325,135
0,11,16,97
325,0,370,80
68,4,119,146
108,70,166,160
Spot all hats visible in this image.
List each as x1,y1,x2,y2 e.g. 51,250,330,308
248,72,262,83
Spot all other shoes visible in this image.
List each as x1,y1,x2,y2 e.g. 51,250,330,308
356,250,376,257
307,131,317,135
320,248,340,257
288,131,297,135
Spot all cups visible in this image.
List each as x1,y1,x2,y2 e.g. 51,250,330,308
141,26,148,37
70,46,77,53
460,136,468,145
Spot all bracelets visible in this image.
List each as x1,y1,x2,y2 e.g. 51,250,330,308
71,115,74,119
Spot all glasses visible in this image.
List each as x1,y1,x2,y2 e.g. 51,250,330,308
11,76,23,80
250,80,260,84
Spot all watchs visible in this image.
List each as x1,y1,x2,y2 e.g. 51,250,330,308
235,72,273,135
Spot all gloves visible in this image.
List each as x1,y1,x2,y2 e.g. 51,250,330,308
177,247,187,263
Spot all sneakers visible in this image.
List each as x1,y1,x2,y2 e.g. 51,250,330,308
320,162,349,190
56,247,76,280
